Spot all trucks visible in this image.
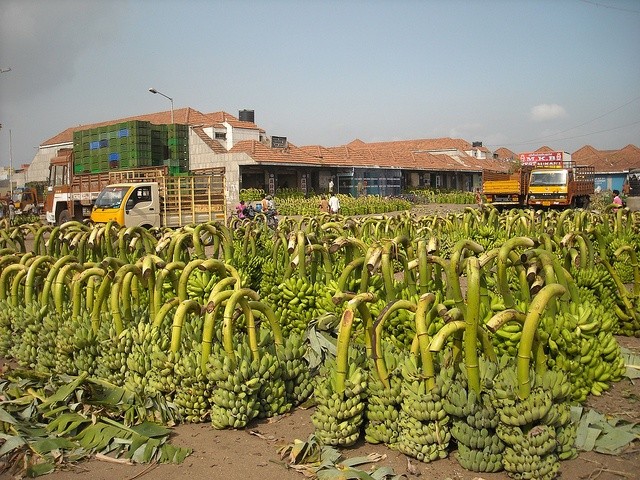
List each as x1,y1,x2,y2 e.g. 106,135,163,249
90,166,227,245
481,167,530,210
528,160,595,208
45,148,169,225
12,187,43,215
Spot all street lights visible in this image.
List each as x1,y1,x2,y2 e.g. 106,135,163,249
149,88,173,123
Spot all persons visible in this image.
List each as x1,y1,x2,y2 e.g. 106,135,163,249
328,177,334,196
260,194,269,213
265,195,277,216
8,200,16,226
236,200,246,219
328,193,341,214
357,179,367,198
320,196,331,213
475,188,480,203
612,190,622,213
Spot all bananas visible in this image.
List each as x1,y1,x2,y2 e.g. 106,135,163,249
0,213,311,430
404,182,478,204
240,186,413,217
314,208,640,401
312,348,578,480
